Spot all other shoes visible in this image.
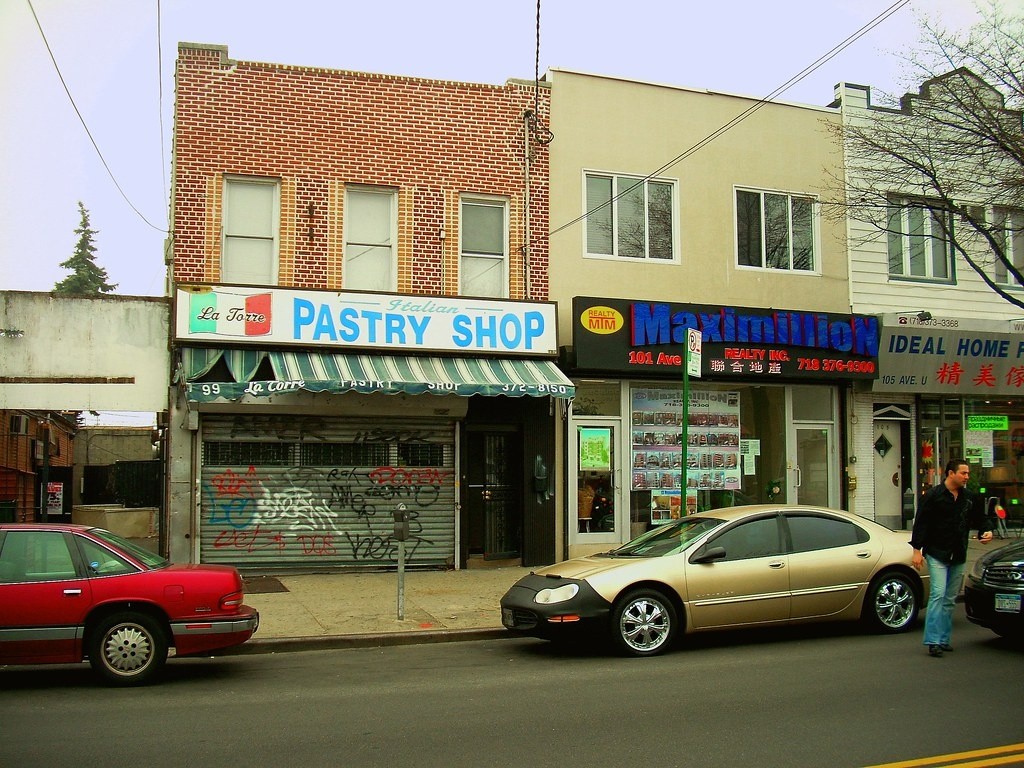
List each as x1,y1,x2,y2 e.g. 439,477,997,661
941,643,953,651
929,644,944,657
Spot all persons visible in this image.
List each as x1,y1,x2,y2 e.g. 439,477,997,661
912,459,993,656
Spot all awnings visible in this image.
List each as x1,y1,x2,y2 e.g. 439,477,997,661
173,349,578,402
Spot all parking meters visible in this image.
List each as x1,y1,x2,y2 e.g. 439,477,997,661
394,502,412,621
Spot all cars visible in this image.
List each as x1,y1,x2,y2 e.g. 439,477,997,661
499,502,933,656
0,524,261,682
963,536,1024,643
